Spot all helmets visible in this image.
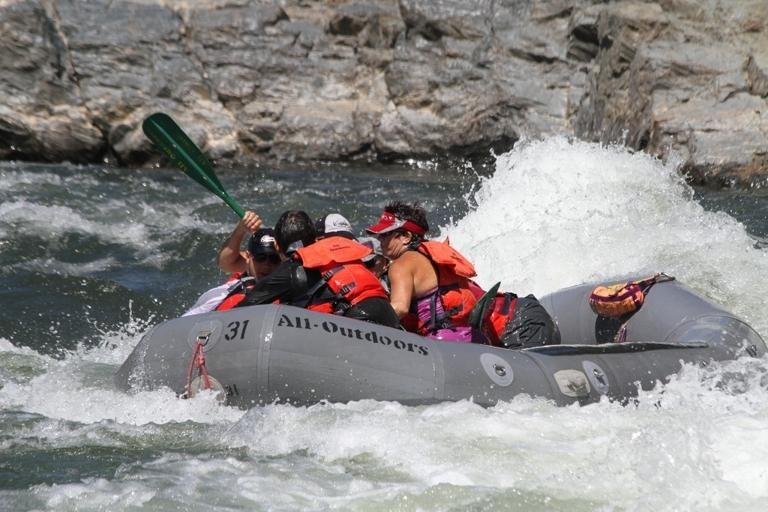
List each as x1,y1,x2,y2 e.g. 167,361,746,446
275,210,314,254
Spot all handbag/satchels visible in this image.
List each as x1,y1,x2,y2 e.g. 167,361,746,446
590,281,644,316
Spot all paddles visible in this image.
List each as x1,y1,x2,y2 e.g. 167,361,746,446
143,112,245,218
469,282,500,331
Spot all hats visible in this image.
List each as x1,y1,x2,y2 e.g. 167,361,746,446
366,212,424,234
315,212,356,239
248,228,279,254
355,237,390,263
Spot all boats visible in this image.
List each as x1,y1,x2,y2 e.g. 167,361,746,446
113,271,768,413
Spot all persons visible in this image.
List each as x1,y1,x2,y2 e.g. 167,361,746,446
233,211,399,328
314,213,356,244
354,236,391,294
210,227,283,312
447,279,562,349
366,201,480,344
217,211,359,275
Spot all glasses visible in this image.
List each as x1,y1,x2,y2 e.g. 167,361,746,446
252,255,280,262
